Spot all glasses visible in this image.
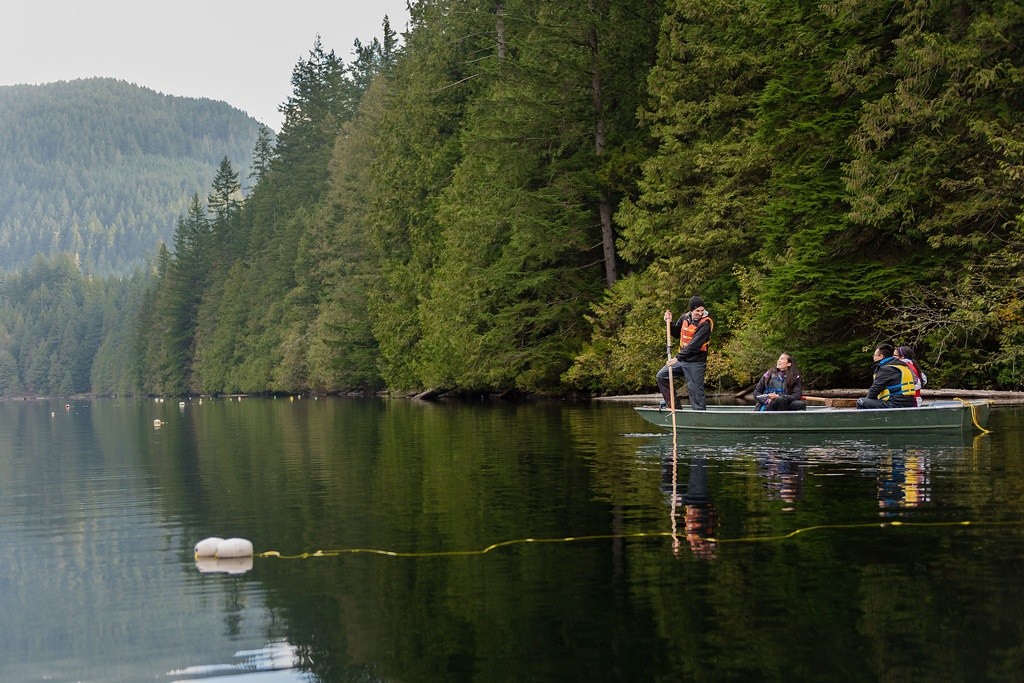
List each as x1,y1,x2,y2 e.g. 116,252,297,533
872,355,879,357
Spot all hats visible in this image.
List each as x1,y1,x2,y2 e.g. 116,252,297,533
689,296,704,312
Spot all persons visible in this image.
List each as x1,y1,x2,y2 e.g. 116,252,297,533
856,344,927,409
656,296,712,410
753,353,807,411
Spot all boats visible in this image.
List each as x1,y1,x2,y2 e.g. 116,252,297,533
632,399,992,437
